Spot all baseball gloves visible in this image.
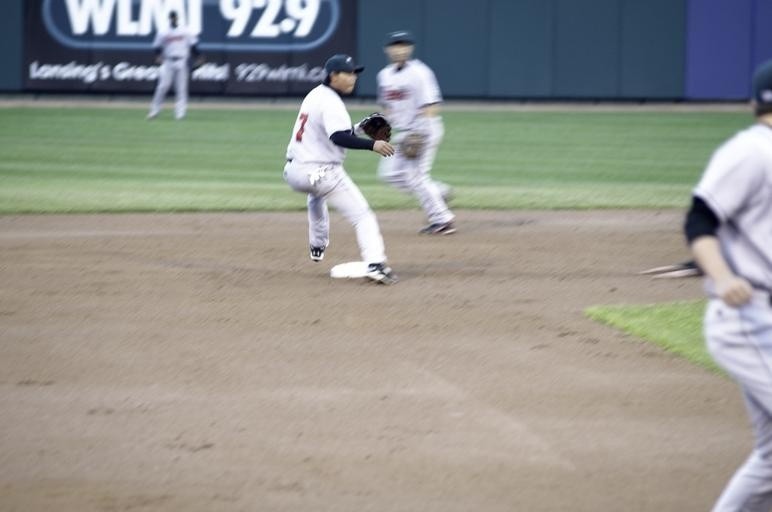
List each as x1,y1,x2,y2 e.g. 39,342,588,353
359,114,391,144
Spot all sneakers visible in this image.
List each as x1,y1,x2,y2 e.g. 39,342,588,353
310,245,325,261
366,264,398,284
420,223,456,235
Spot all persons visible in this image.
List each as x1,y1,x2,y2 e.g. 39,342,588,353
144,9,201,121
374,28,460,237
681,58,771,512
281,51,399,285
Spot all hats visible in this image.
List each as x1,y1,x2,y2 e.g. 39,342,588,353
326,54,364,72
384,31,414,43
